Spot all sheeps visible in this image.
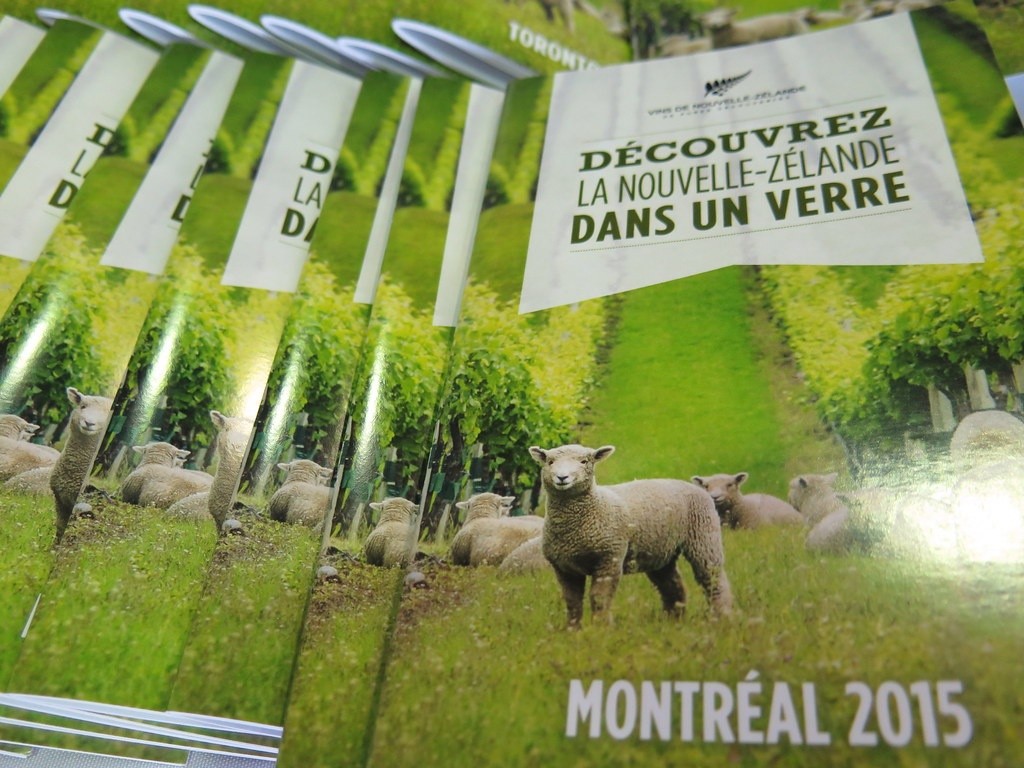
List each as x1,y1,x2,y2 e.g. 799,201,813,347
649,0,936,58
208,410,254,535
537,0,599,32
4,468,55,497
268,459,333,527
0,415,61,475
528,445,730,629
449,492,543,566
161,492,213,523
49,387,113,544
691,474,804,528
364,497,420,570
788,473,881,551
120,441,214,508
500,535,552,570
